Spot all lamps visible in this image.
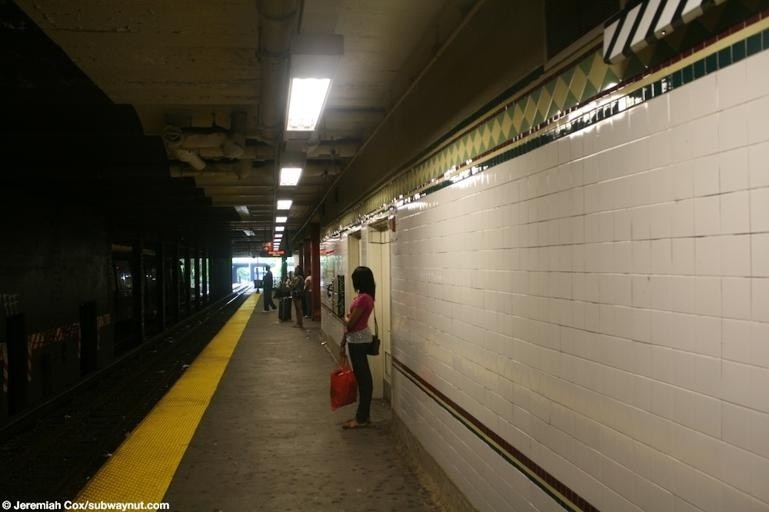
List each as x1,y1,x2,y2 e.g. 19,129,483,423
270,29,345,252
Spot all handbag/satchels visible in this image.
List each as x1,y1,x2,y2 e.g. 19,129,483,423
330,357,357,411
367,335,380,356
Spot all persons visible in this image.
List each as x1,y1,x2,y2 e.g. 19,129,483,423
303,269,313,319
339,265,377,429
261,265,278,313
274,271,295,299
291,265,305,329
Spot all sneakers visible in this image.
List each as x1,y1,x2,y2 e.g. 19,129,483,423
343,415,371,429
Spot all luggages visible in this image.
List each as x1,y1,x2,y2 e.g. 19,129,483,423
279,291,292,321
